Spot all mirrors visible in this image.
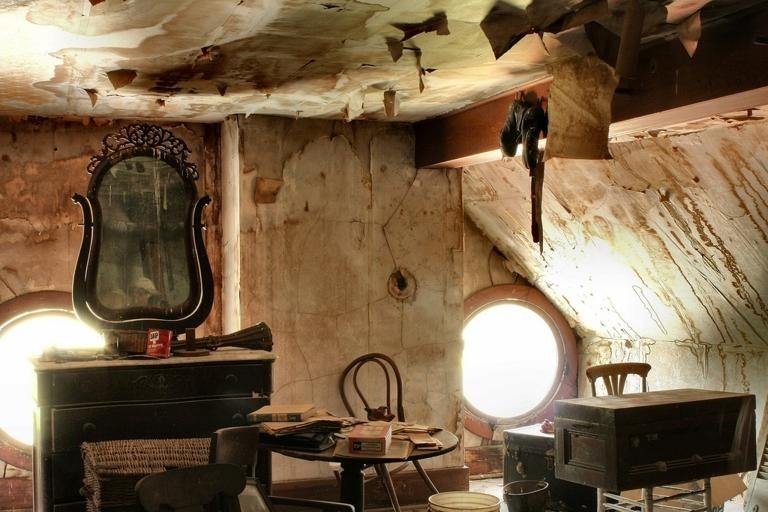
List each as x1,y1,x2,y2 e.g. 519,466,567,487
70,122,216,331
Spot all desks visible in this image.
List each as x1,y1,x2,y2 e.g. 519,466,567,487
268,419,459,512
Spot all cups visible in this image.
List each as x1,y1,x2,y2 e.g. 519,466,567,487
146,328,173,358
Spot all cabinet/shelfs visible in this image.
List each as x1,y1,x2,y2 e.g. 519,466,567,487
28,346,277,511
553,388,757,493
502,420,620,512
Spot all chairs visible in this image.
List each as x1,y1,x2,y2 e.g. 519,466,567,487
333,352,439,511
134,462,247,511
202,424,355,511
585,362,712,512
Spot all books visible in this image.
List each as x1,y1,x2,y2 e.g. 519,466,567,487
246,405,316,423
348,420,444,455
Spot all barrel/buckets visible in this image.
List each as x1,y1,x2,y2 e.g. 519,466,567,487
502,480,550,512
428,491,501,512
